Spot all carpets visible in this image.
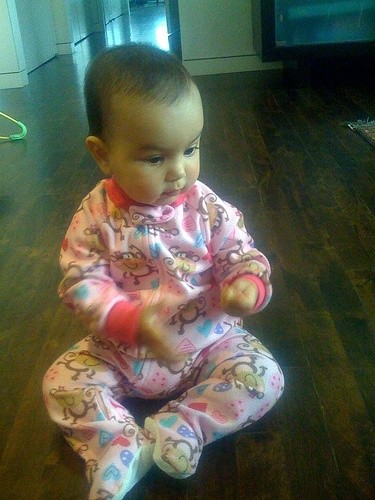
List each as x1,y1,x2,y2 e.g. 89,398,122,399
346,116,375,150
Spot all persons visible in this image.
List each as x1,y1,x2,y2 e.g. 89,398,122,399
41,41,286,500
